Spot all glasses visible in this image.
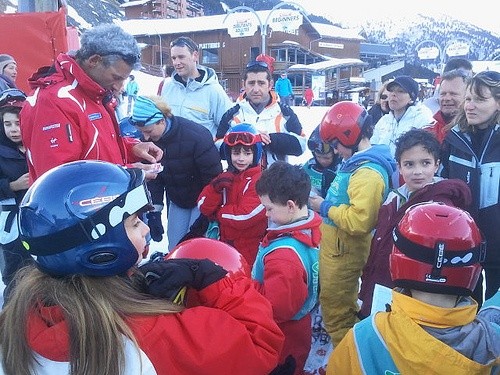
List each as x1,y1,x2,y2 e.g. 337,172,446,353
0,90,26,101
380,94,388,100
88,52,141,64
244,61,269,69
308,141,333,154
223,132,261,146
170,40,195,51
128,112,165,126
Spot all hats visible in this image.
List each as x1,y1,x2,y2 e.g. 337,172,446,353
387,76,418,105
0,54,16,75
0,88,27,107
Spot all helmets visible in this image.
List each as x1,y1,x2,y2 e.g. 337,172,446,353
389,201,486,294
19,160,148,277
165,238,251,305
118,117,143,139
320,102,368,148
222,124,263,165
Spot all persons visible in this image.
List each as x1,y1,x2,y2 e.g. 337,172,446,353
275,71,295,107
433,75,441,87
251,59,500,375
362,90,371,109
0,22,285,375
306,86,315,109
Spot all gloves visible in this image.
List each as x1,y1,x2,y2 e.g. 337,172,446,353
208,209,219,221
271,355,296,375
292,94,294,99
210,173,234,193
142,259,228,298
